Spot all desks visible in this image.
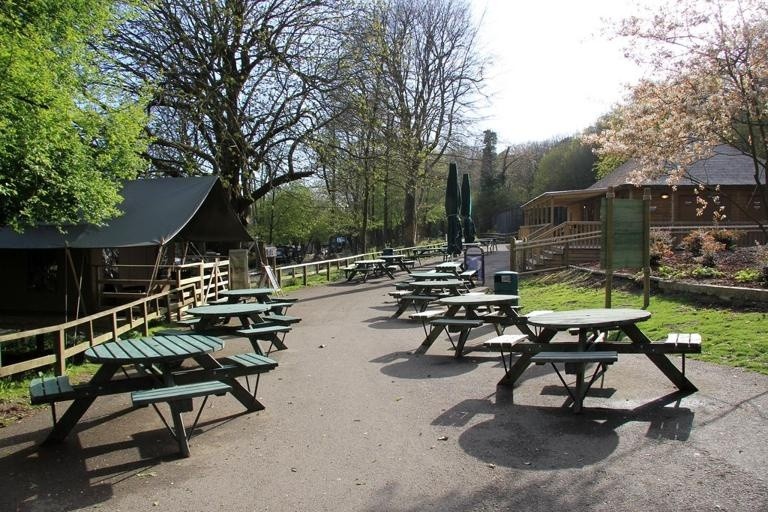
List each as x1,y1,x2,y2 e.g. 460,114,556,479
524,307,652,354
408,261,463,287
344,254,415,282
462,238,498,255
439,294,528,325
413,245,448,262
83,334,226,396
185,288,275,317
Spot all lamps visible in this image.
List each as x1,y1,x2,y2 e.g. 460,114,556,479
430,317,485,360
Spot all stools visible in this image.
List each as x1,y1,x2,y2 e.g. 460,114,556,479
515,310,554,342
131,380,233,459
236,296,302,356
29,374,97,447
528,350,619,414
152,319,201,335
226,352,278,412
387,283,438,319
487,305,524,337
458,269,489,293
481,334,533,387
650,332,702,394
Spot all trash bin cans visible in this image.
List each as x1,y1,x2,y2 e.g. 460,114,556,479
493,270,519,314
382,248,393,266
